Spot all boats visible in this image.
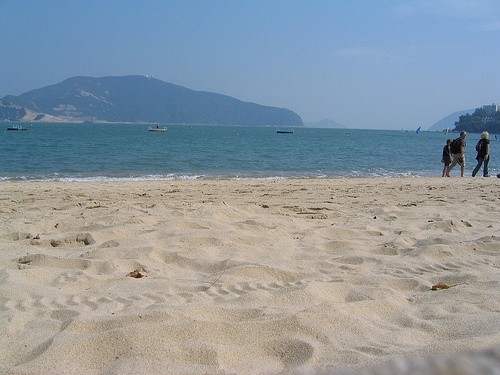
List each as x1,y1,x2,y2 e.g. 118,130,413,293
148,127,168,132
276,130,295,134
6,126,28,130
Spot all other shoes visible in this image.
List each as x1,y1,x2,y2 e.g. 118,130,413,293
446,173,450,177
484,174,489,177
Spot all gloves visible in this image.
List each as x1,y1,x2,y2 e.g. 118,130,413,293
484,155,488,161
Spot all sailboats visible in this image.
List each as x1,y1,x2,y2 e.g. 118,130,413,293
415,126,422,134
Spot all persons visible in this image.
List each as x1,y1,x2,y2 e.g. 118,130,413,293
442,139,451,177
471,131,490,177
446,131,466,177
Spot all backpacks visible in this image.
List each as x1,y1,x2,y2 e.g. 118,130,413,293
450,139,462,154
476,139,485,151
